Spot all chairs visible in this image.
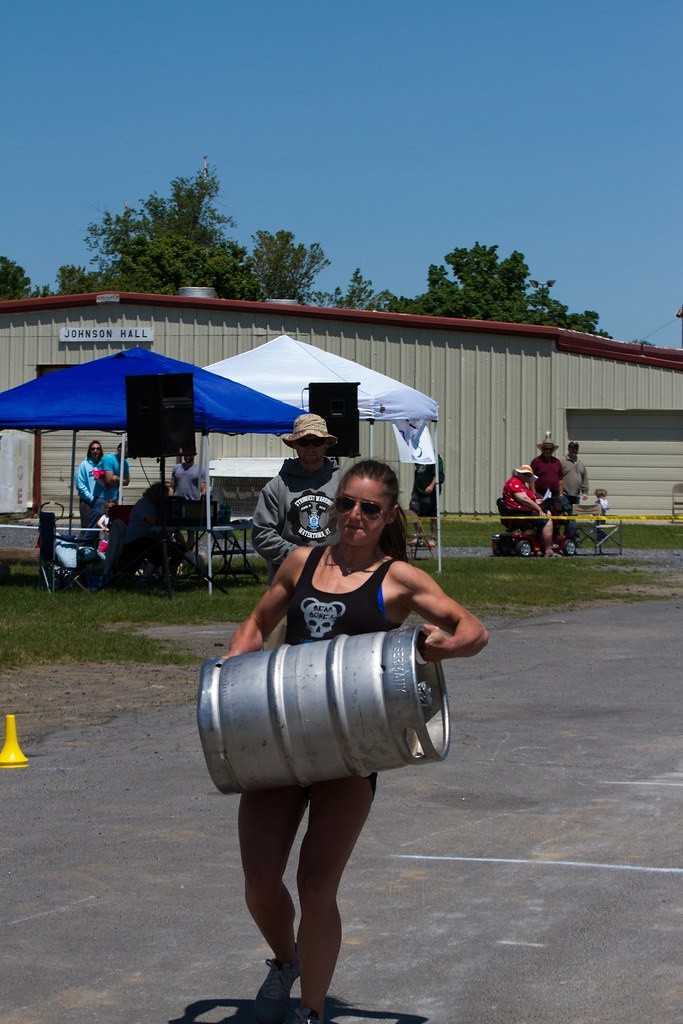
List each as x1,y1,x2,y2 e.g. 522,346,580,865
404,519,436,560
572,505,623,555
671,483,683,522
37,512,102,596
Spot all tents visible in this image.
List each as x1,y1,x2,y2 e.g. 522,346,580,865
0,348,310,594
195,334,441,572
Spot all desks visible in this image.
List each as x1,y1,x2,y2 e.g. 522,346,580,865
150,516,259,597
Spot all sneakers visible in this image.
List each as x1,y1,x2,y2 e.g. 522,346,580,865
252,942,300,1024
291,1008,325,1024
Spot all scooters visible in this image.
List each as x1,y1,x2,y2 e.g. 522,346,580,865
490,495,578,557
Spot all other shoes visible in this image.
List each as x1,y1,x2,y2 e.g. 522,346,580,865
407,538,426,547
426,540,436,547
543,552,562,558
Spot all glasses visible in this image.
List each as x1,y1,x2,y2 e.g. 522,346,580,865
524,473,531,478
334,494,390,521
91,447,100,451
544,448,554,452
298,437,327,448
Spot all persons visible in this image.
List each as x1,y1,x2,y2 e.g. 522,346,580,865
594,489,608,523
168,455,206,554
560,442,589,542
502,465,562,557
529,439,564,516
220,461,490,1024
75,440,129,548
251,414,344,651
123,484,178,588
407,450,445,547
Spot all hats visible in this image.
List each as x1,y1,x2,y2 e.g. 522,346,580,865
536,438,561,453
513,464,539,483
567,441,579,450
279,413,338,449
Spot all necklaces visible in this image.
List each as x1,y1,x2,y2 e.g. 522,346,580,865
336,543,380,573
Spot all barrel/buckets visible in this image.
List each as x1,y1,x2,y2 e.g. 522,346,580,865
195,624,450,792
